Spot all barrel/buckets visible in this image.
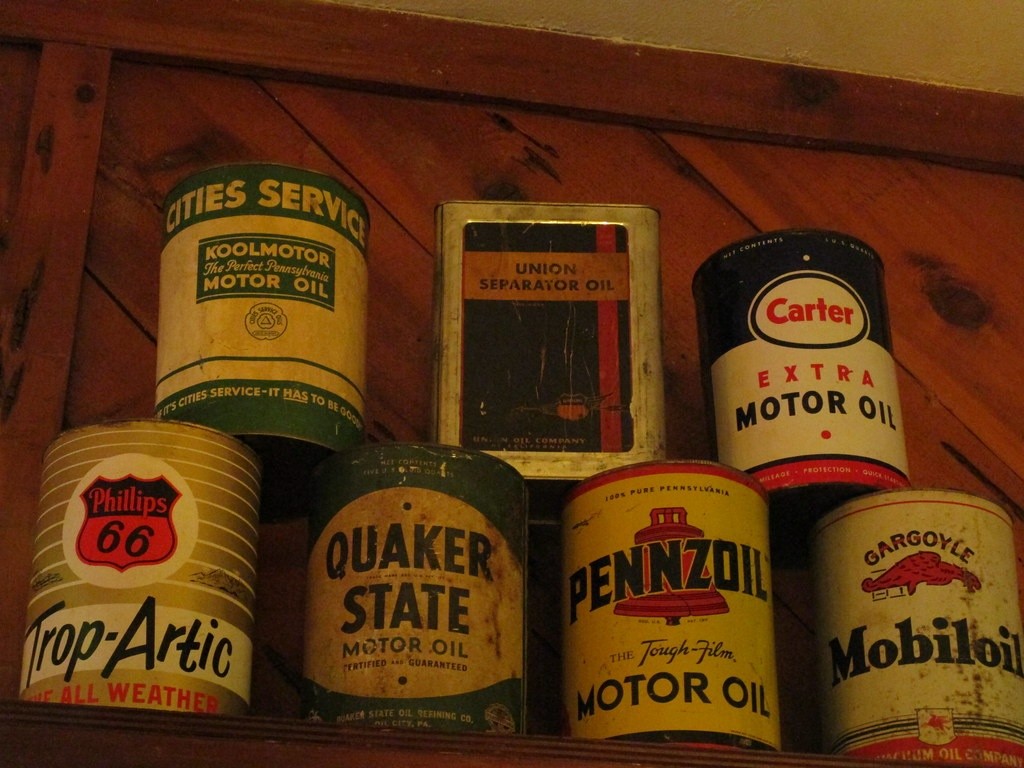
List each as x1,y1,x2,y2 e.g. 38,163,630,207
561,457,783,755
811,487,1023,768
154,163,370,463
689,226,911,500
300,442,533,738
14,416,268,722
